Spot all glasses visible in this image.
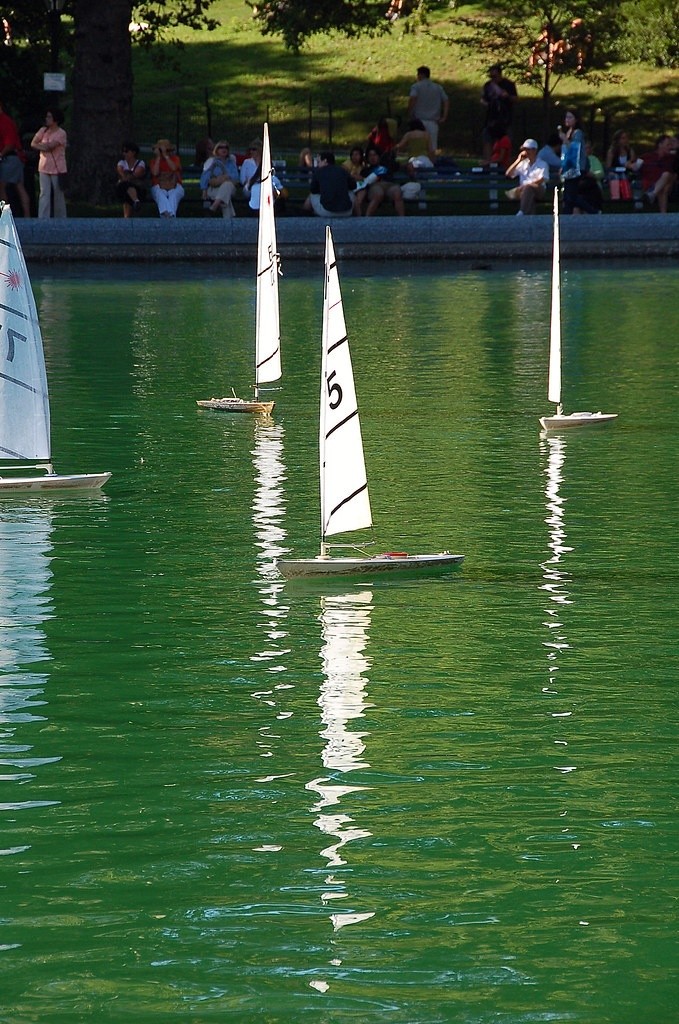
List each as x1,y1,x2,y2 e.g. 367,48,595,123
122,150,129,153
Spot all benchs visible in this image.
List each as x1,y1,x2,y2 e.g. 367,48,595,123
141,165,679,216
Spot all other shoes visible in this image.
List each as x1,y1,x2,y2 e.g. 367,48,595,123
209,205,217,213
134,200,140,210
644,193,655,203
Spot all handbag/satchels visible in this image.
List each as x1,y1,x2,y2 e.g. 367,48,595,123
620,173,632,200
59,173,69,191
159,171,176,189
609,174,620,201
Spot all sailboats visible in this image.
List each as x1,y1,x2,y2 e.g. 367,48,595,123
195,117,283,414
537,184,620,431
0,199,116,493
271,224,467,579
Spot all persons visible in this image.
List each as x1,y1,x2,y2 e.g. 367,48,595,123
200,140,239,218
117,143,146,218
2,18,13,46
298,149,318,182
239,139,287,216
479,66,518,167
342,147,405,216
367,119,434,157
149,140,185,217
405,66,448,151
0,103,30,217
385,0,405,22
303,152,360,216
506,139,549,215
31,109,67,217
538,109,679,213
525,18,593,80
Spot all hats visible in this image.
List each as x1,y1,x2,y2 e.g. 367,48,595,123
520,138,538,149
152,139,176,154
